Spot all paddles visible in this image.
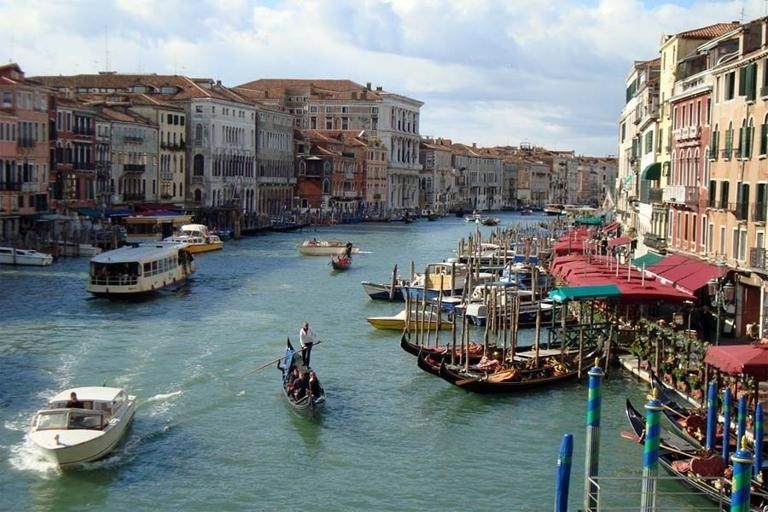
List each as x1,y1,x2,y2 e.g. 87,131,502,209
620,431,701,460
646,395,687,418
455,370,514,385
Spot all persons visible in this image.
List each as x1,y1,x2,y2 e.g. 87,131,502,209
300,321,317,369
460,339,570,383
345,241,352,257
64,391,84,416
14,214,107,251
287,370,309,400
306,371,322,396
289,369,298,384
95,263,138,284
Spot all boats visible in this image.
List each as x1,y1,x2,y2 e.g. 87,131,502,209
279,338,326,409
30,386,137,468
624,387,768,512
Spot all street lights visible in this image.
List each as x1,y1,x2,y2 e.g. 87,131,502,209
707,277,735,346
